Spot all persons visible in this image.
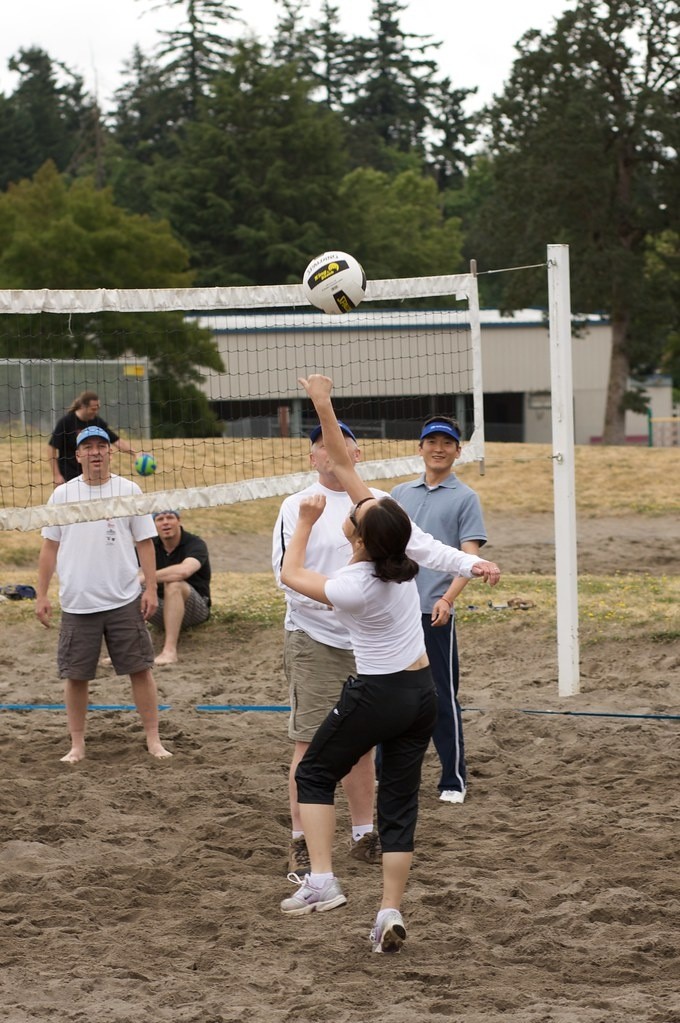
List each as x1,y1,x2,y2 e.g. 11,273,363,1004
272,419,501,877
104,511,212,664
281,374,438,955
34,425,173,764
48,392,141,487
390,416,488,803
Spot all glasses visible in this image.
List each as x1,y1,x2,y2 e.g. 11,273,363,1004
349,496,376,537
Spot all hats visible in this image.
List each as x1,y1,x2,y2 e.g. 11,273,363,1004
151,510,179,521
76,426,110,447
420,421,460,446
310,419,356,445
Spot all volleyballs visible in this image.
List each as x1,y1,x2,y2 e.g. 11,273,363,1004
135,454,157,477
302,249,368,316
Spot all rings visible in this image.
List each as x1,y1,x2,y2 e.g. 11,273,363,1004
494,568,498,570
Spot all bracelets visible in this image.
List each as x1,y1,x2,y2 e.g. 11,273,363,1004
441,597,451,608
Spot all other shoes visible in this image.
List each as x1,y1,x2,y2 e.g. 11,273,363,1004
375,780,379,792
438,789,466,803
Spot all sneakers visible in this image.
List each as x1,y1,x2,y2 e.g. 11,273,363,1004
347,829,383,863
280,872,347,917
287,835,310,875
369,908,407,953
